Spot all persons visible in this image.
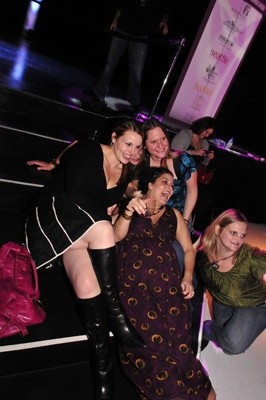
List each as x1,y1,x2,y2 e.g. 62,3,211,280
25,116,266,400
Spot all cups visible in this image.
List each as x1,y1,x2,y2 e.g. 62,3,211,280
144,199,156,218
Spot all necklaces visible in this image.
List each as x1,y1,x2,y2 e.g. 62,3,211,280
146,206,163,215
212,247,233,269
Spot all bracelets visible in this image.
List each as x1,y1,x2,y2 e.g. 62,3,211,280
125,207,133,213
184,219,189,223
121,212,132,220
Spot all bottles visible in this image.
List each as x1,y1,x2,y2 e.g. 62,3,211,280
226,137,234,149
